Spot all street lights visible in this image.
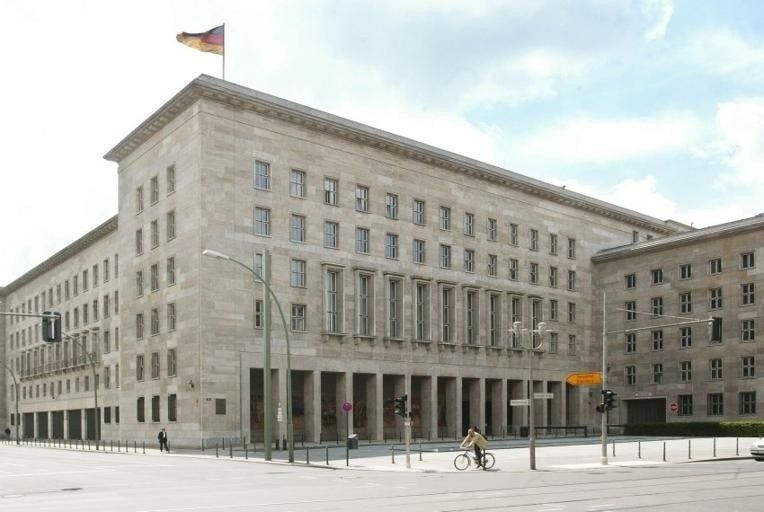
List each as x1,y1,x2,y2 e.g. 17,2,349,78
202,248,295,463
61,331,98,450
512,319,547,470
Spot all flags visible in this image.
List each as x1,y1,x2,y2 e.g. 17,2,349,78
176,24,224,57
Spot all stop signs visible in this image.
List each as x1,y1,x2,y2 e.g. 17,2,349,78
669,403,678,412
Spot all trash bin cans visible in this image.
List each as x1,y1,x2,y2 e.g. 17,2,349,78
348,433,358,449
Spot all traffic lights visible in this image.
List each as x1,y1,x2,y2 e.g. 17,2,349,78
604,389,618,411
596,405,603,412
393,394,407,418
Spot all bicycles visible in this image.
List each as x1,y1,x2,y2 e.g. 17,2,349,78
453,447,495,471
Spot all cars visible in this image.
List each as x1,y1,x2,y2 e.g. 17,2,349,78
750,436,764,462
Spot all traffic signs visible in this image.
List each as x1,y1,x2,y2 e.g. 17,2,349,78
532,392,553,400
509,399,532,407
565,371,601,386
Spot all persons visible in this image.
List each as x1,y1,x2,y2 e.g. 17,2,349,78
460,428,488,471
158,427,169,452
3,428,11,440
